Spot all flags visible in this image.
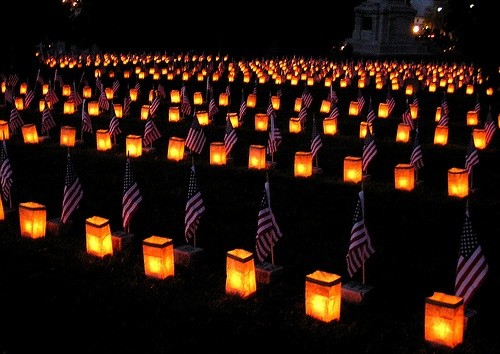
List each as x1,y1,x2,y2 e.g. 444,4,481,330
455,208,489,310
122,158,143,228
255,181,283,263
184,165,206,243
0,68,500,177
346,190,376,277
61,154,84,224
0,139,14,202
